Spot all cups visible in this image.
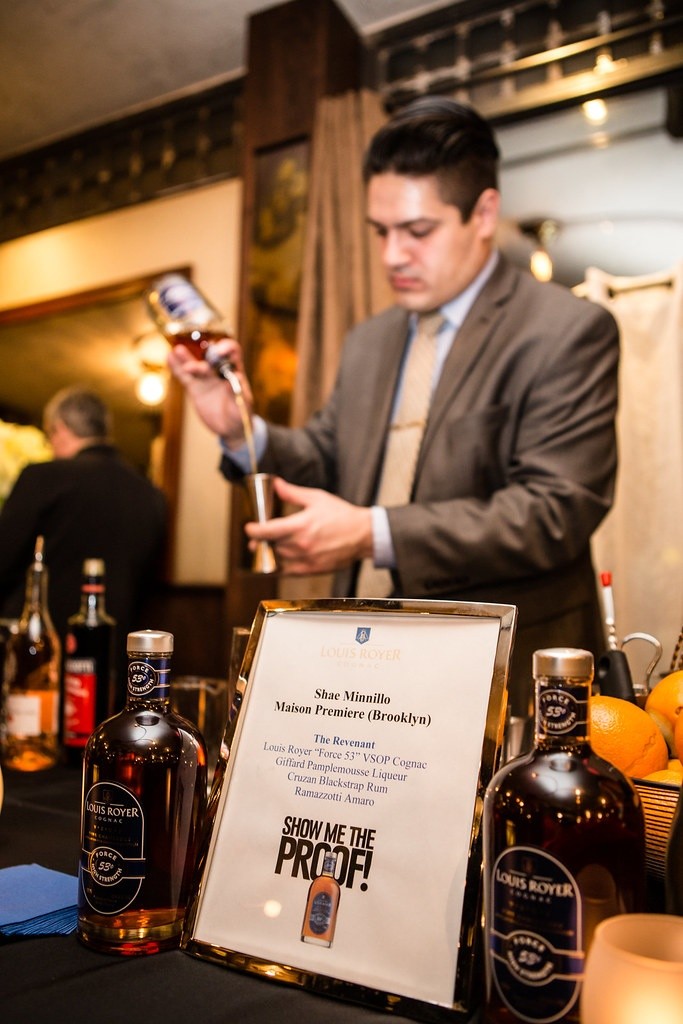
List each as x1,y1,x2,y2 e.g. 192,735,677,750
244,471,278,575
579,912,683,1024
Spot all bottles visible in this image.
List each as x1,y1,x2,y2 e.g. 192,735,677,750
2,553,61,772
482,646,644,1024
143,272,243,396
76,630,209,957
300,852,341,948
59,559,120,771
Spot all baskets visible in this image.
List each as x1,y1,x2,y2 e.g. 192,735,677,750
623,774,681,890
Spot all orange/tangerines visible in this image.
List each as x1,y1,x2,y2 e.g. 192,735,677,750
589,671,683,867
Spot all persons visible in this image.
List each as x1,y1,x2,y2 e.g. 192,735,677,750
1,388,175,710
172,97,622,722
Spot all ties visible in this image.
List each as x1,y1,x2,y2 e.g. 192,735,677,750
355,310,445,597
